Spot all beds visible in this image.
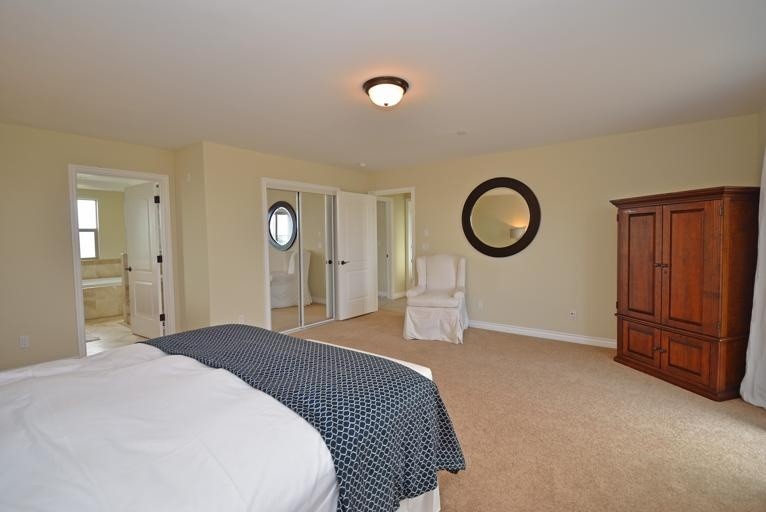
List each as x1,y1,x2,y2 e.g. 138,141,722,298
0,323,465,511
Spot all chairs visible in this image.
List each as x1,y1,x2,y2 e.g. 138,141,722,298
270,248,312,308
403,257,469,346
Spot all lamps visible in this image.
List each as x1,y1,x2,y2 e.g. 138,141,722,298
362,78,408,107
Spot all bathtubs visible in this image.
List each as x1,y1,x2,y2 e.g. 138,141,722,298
82,277,122,319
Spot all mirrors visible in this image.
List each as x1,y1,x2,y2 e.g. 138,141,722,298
265,179,333,334
267,201,298,250
462,180,539,256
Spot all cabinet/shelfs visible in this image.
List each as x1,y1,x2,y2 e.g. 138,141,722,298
609,186,760,401
